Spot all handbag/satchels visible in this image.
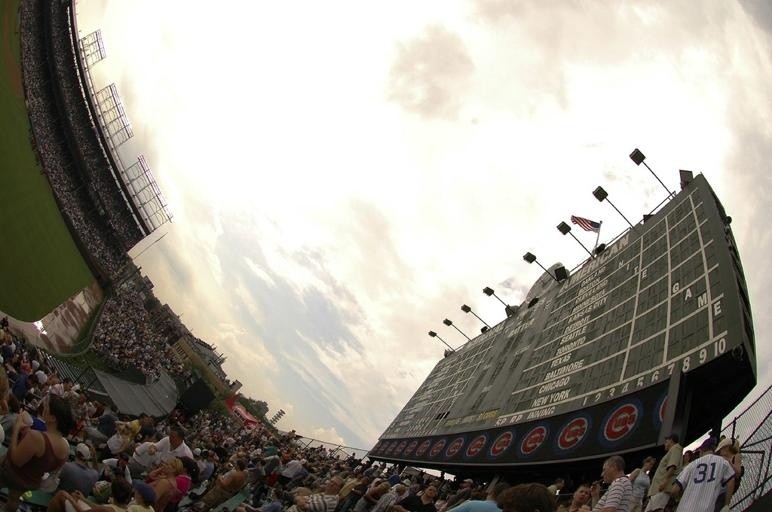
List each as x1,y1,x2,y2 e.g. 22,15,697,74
41,471,60,495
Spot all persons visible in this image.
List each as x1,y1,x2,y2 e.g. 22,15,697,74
1,0,743,512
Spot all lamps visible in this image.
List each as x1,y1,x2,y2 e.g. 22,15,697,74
443,319,470,340
483,287,514,313
429,331,454,350
593,186,633,228
523,252,556,280
629,148,674,198
461,305,491,328
557,221,591,255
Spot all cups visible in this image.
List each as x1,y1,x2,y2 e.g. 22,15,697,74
18,410,34,431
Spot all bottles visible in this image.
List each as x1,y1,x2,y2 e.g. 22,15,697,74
21,491,34,501
200,479,208,493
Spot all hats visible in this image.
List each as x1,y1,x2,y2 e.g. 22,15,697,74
76,443,90,460
133,478,157,503
